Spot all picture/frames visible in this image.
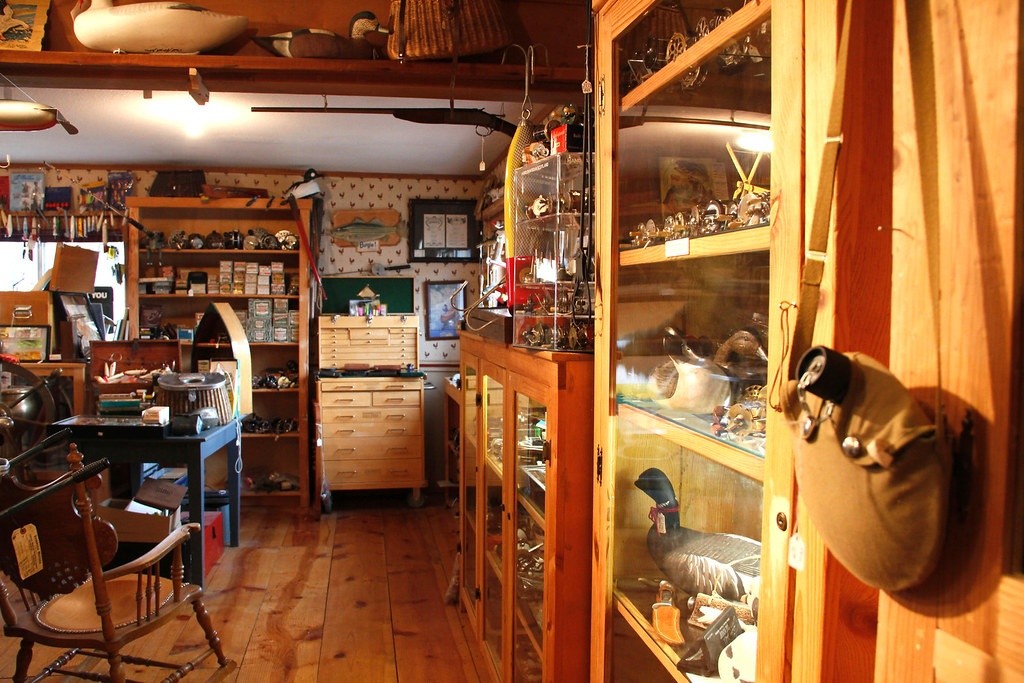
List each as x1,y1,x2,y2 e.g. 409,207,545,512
425,280,466,341
0,325,51,363
0,0,51,51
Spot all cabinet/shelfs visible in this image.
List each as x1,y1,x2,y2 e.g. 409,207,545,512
0,0,1024,683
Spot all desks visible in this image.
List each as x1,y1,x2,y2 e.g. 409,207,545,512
52,413,250,585
15,361,86,484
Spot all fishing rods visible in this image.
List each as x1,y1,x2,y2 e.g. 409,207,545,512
42,159,156,241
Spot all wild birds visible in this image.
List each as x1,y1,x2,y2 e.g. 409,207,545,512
634,468,761,604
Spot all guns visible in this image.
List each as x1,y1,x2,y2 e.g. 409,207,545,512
248,103,520,139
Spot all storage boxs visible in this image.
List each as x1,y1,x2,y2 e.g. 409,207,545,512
181,510,224,577
0,243,99,353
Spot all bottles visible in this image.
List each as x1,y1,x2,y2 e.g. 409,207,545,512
140,228,224,249
522,144,531,165
143,247,156,278
157,251,167,277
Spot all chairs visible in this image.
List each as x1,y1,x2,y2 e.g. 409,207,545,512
0,429,237,683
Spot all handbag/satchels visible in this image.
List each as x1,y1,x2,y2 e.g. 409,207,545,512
786,346,947,593
148,168,206,198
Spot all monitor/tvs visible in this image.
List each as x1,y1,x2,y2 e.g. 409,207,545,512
0,325,51,362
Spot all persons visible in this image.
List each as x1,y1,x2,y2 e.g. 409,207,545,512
30,182,41,203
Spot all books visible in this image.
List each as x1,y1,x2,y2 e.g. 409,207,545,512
123,478,188,517
97,394,141,418
